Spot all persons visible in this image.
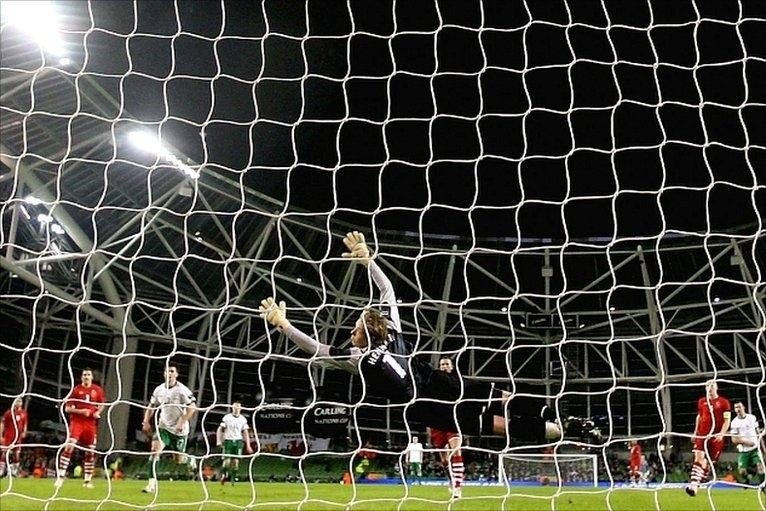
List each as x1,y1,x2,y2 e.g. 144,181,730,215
1,432,370,484
424,354,466,504
142,364,198,496
684,380,732,499
0,397,28,477
730,400,766,494
215,401,254,485
54,368,107,490
257,230,610,455
395,432,736,490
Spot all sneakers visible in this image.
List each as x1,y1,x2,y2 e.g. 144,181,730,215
686,482,698,498
143,484,156,494
562,414,624,444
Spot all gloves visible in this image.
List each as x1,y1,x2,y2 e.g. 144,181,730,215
259,297,286,326
342,231,369,266
